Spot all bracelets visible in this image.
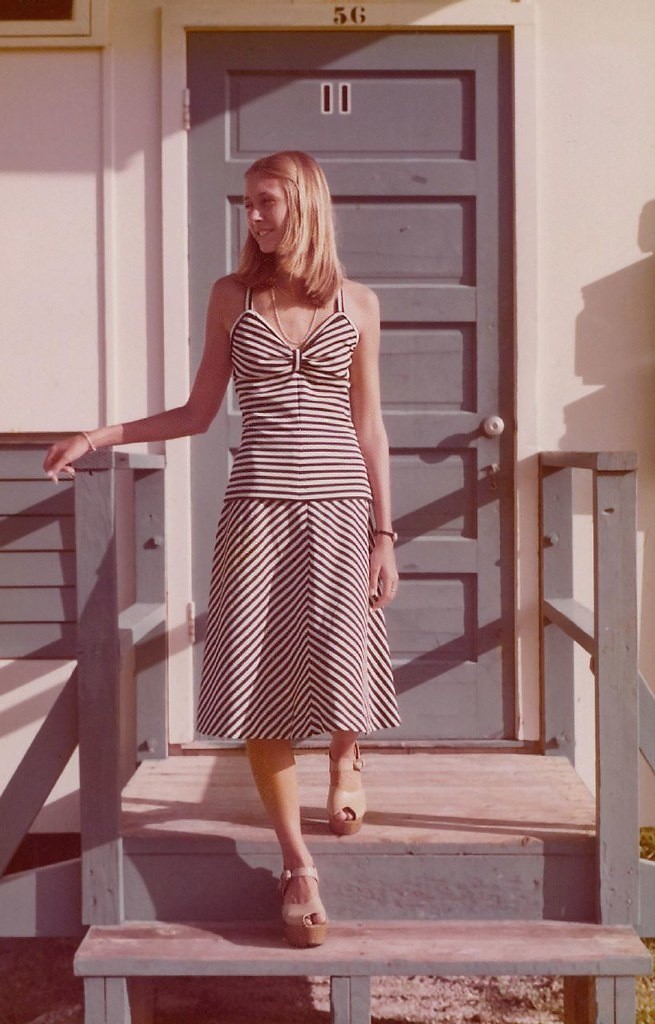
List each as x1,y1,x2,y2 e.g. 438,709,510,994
374,529,398,544
81,431,97,452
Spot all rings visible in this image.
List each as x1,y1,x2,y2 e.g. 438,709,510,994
391,588,396,592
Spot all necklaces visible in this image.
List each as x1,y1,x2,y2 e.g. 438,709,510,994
270,284,318,344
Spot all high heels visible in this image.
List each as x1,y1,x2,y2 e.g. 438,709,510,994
326,736,367,834
279,863,328,947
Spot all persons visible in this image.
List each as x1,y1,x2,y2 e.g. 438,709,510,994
42,150,400,947
